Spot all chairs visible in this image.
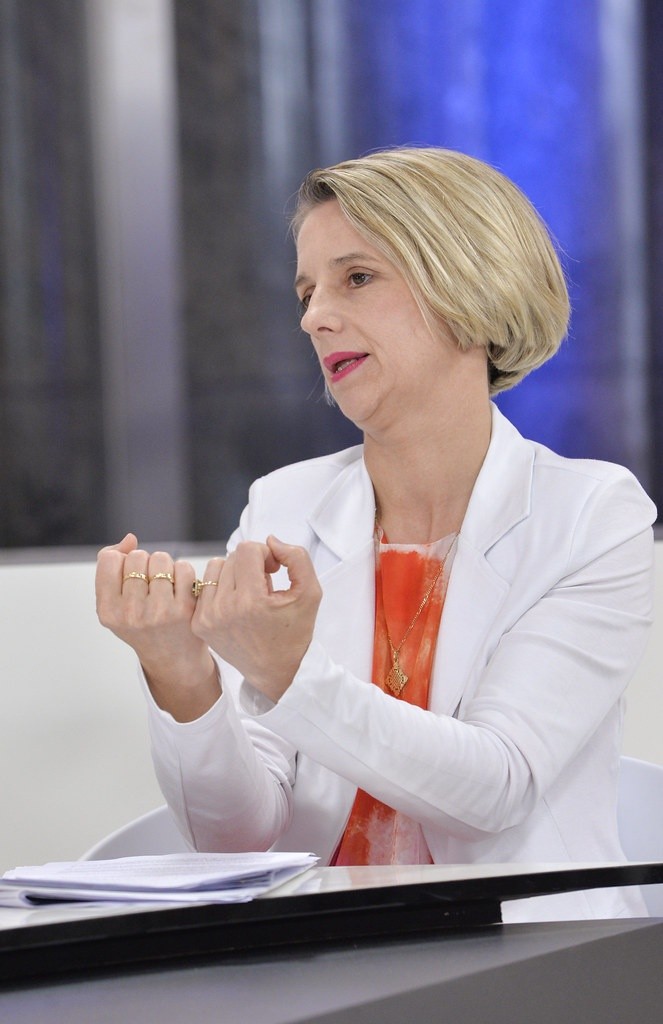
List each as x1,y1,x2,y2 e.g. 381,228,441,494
82,755,663,917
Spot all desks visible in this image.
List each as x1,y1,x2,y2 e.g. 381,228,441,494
0,862,663,1024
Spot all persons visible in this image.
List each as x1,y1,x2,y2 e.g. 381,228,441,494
95,147,657,925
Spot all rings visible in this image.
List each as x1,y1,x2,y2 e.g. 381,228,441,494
123,572,149,584
150,572,175,585
191,579,218,597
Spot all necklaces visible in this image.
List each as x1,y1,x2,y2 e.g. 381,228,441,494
374,503,460,698
